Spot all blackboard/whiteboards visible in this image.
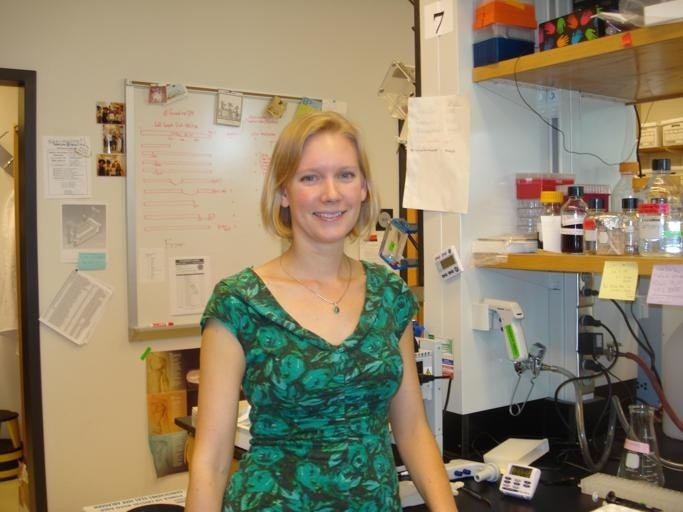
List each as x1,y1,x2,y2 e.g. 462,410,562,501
124,76,324,343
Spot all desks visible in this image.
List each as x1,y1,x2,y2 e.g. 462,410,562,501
174,405,682,512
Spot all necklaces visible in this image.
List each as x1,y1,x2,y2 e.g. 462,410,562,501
278,252,353,315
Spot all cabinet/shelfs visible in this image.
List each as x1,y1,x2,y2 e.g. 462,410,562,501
468,16,682,280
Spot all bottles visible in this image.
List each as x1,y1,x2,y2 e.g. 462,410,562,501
616,403,667,489
514,156,683,258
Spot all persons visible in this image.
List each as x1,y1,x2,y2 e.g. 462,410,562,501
184,112,458,511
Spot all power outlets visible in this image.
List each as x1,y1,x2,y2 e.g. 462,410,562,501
578,272,597,397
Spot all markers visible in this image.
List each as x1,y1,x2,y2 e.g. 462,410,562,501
150,322,175,328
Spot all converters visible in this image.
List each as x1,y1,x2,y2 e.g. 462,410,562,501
578,333,603,355
